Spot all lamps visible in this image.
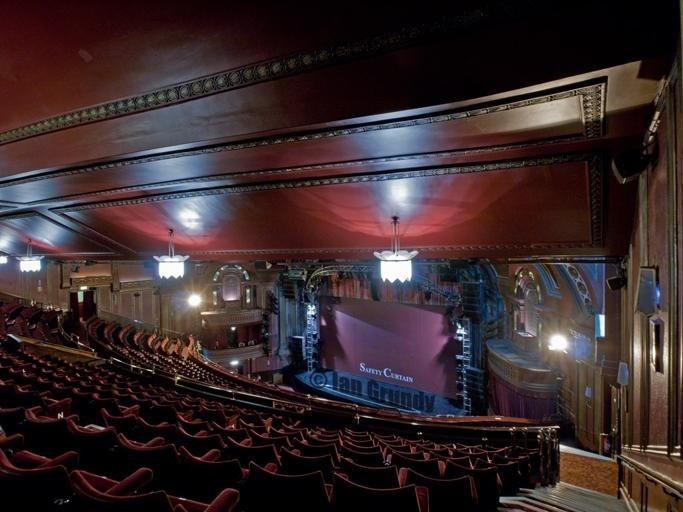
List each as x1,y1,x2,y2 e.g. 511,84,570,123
373,216,419,283
15,239,45,272
153,229,190,279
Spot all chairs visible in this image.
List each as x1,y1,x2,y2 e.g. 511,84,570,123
0,300,539,512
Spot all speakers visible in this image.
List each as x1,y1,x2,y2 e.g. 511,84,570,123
610,147,648,185
606,276,626,291
